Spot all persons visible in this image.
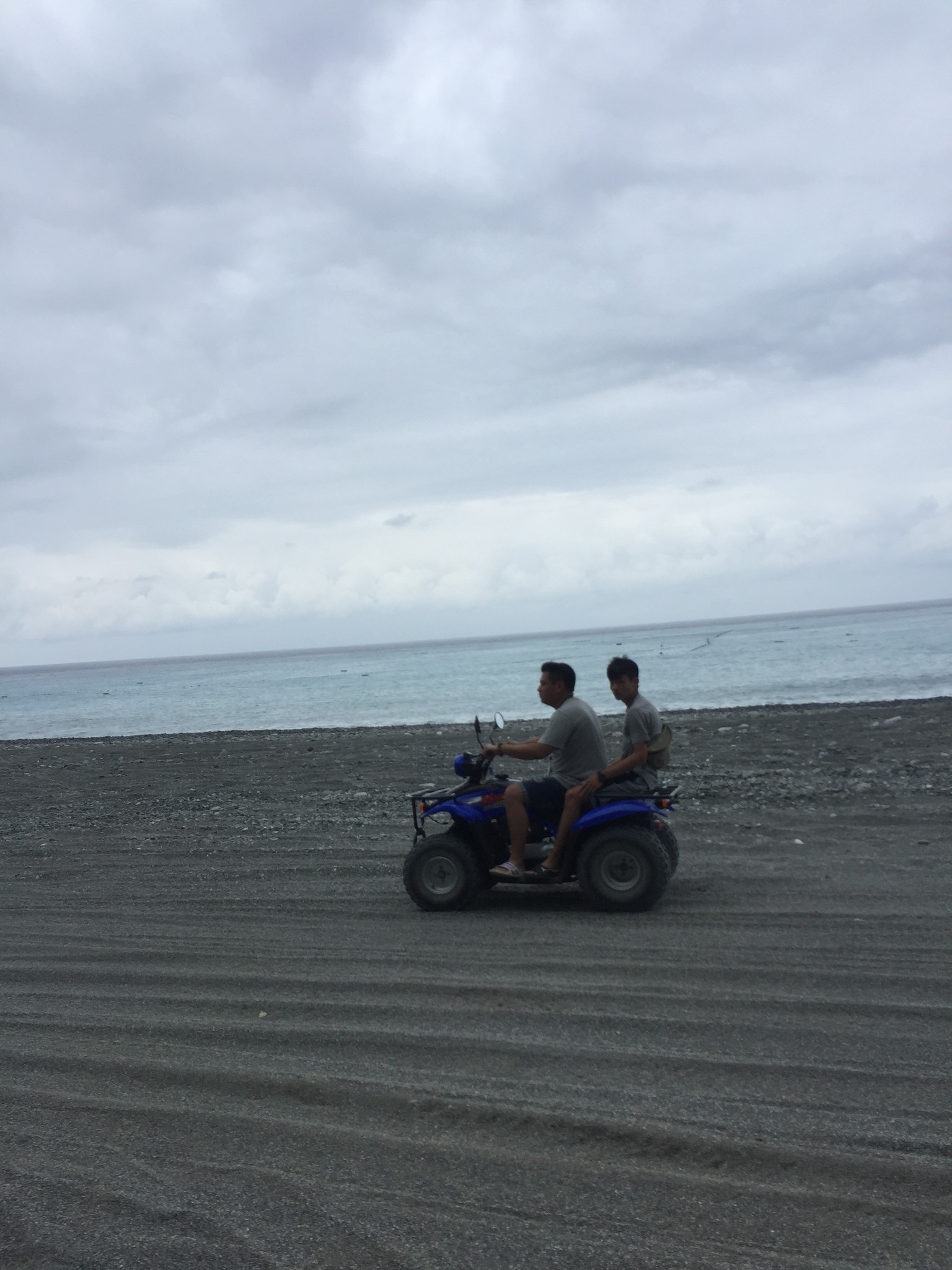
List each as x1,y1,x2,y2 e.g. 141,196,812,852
524,655,663,883
481,662,608,878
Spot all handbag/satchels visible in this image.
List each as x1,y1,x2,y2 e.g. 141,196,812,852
646,724,672,770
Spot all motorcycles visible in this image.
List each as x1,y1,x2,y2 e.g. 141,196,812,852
403,712,680,912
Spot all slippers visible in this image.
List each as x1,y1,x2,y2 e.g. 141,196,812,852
524,863,560,878
489,862,525,877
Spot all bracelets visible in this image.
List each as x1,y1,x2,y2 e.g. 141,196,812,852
496,742,503,757
598,770,608,783
